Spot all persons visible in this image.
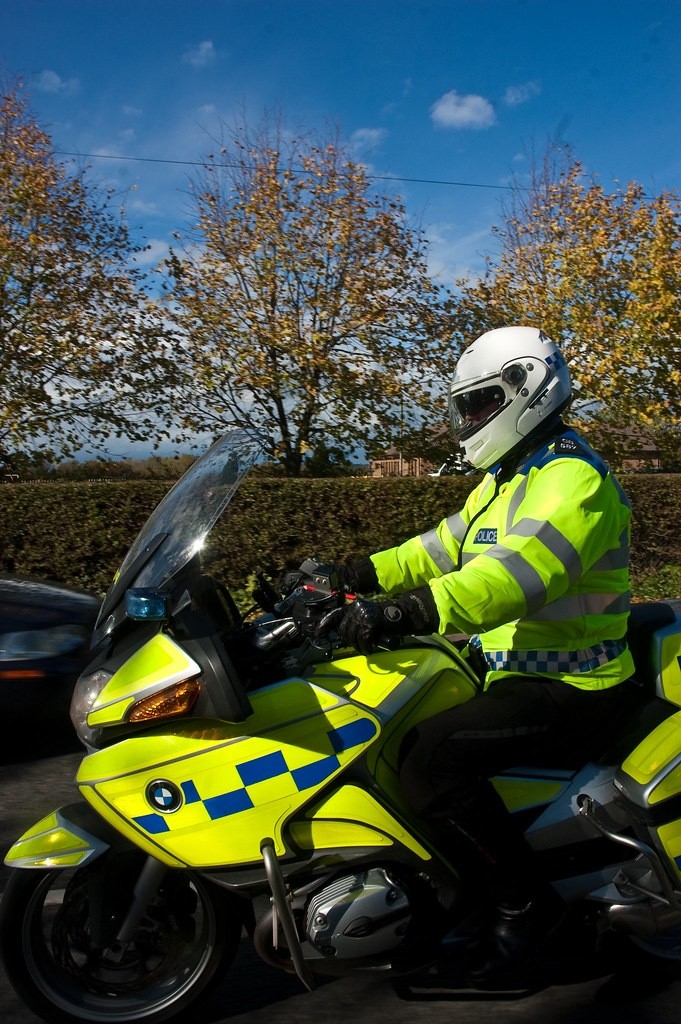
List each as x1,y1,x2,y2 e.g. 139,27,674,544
282,325,650,992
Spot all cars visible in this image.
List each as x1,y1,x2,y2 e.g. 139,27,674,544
0,578,102,789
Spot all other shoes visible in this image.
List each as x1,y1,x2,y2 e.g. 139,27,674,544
468,876,565,982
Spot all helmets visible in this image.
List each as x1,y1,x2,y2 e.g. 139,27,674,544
450,326,572,473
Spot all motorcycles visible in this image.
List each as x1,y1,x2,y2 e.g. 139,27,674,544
0,446,680,1024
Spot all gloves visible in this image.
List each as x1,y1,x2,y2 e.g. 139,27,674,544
313,554,378,594
316,585,440,657
